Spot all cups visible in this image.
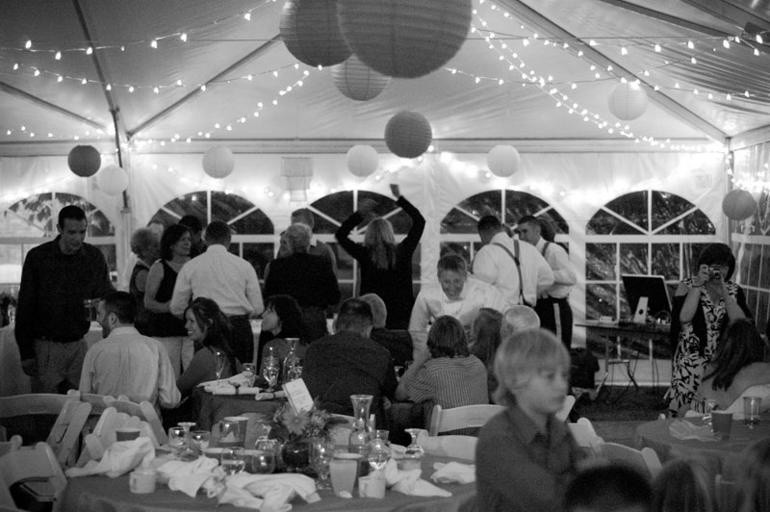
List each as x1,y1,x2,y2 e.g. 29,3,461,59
242,364,255,393
359,475,386,501
330,459,359,496
310,444,334,489
129,471,156,494
335,454,359,486
262,358,279,395
251,448,277,473
214,354,224,390
744,397,759,429
711,411,729,440
221,450,243,479
84,298,96,323
368,440,390,477
169,427,184,462
224,417,248,448
189,431,210,466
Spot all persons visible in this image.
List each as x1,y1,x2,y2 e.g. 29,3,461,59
254,297,313,388
692,319,770,417
335,183,428,330
515,215,577,348
500,306,539,343
145,218,167,243
262,229,288,284
175,214,209,257
127,230,159,295
289,209,338,274
468,309,516,364
171,220,266,364
261,223,342,342
355,294,415,377
295,301,397,423
79,289,183,422
664,242,756,420
394,315,490,436
15,206,119,395
561,460,659,512
473,214,557,309
300,297,398,435
475,329,589,512
144,225,193,338
177,297,250,421
408,251,510,353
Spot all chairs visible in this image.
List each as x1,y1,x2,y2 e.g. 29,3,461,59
568,417,604,449
429,403,507,436
69,388,129,430
0,436,21,460
0,386,78,468
105,400,168,445
327,413,378,428
23,388,93,471
590,436,661,480
556,395,578,422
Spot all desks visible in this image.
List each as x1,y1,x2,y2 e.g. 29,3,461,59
194,375,424,432
635,416,770,512
575,316,673,411
53,440,478,511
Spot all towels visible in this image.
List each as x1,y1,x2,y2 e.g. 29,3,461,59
211,381,261,397
157,454,225,499
365,457,454,500
214,471,317,512
253,390,288,402
430,460,477,486
61,435,156,481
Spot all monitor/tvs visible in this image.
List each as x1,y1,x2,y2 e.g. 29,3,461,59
620,273,673,326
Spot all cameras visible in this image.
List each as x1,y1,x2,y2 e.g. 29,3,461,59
707,268,722,279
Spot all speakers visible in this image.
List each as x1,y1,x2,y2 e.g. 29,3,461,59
570,347,600,389
